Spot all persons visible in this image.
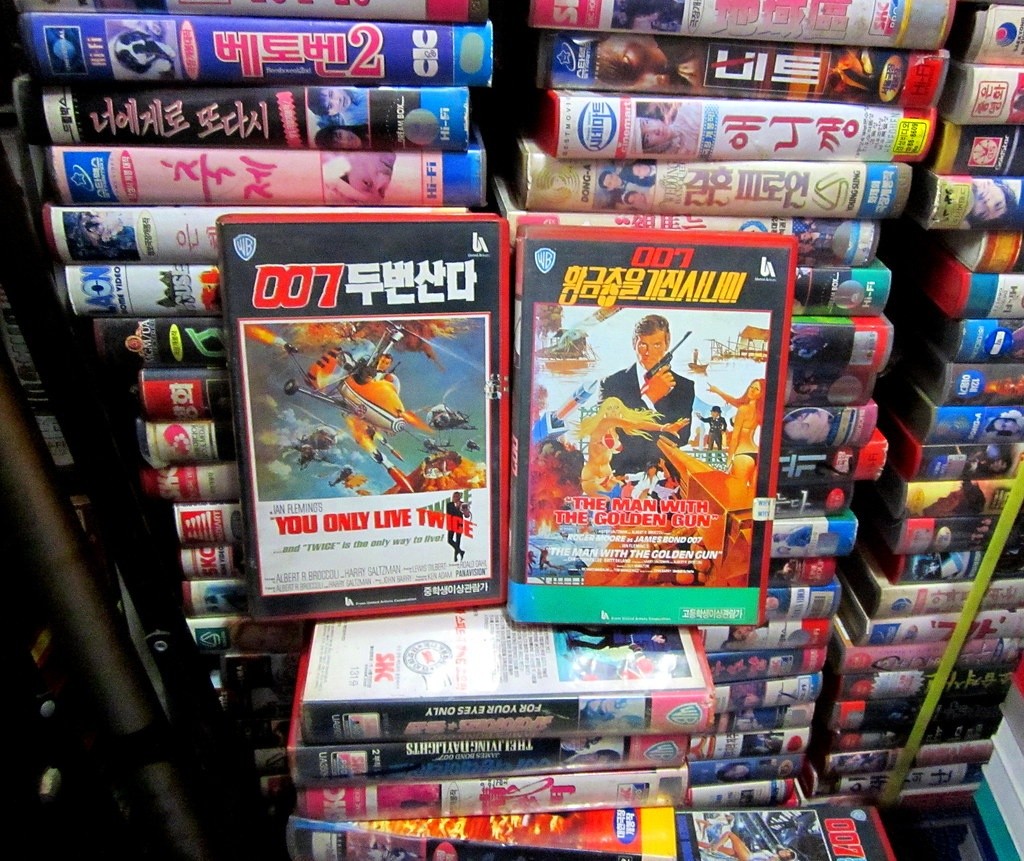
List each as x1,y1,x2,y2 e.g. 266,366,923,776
631,161,657,181
785,412,830,445
306,89,371,122
599,171,625,195
772,526,813,546
311,125,371,149
447,487,465,561
697,830,795,860
629,104,699,156
731,626,768,642
600,314,694,498
984,417,1021,437
591,34,678,93
715,762,776,781
359,354,392,380
969,178,1018,233
626,190,654,211
778,547,807,558
322,152,396,204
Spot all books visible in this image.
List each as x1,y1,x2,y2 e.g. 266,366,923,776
0,0,1024,860
